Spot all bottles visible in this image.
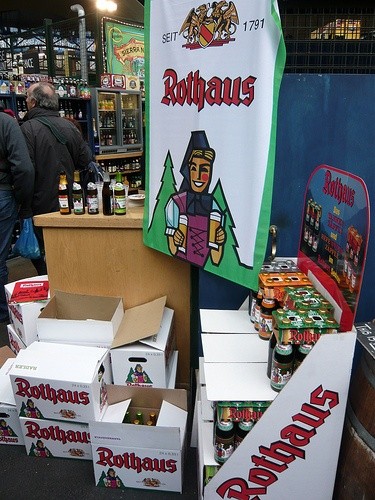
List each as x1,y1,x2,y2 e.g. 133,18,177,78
124,408,158,427
58,170,71,215
340,224,362,294
72,170,85,215
0,98,83,121
247,259,339,393
212,418,255,464
114,171,127,216
303,198,321,253
86,171,99,214
92,91,143,188
0,60,91,98
102,172,115,215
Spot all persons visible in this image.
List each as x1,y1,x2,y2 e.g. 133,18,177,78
18,82,96,276
1,101,35,348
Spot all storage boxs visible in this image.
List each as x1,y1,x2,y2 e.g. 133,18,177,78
0,275,189,494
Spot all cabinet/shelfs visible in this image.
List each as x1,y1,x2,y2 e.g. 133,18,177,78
0,78,146,191
195,165,370,500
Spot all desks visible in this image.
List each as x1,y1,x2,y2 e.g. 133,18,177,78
33,198,189,384
